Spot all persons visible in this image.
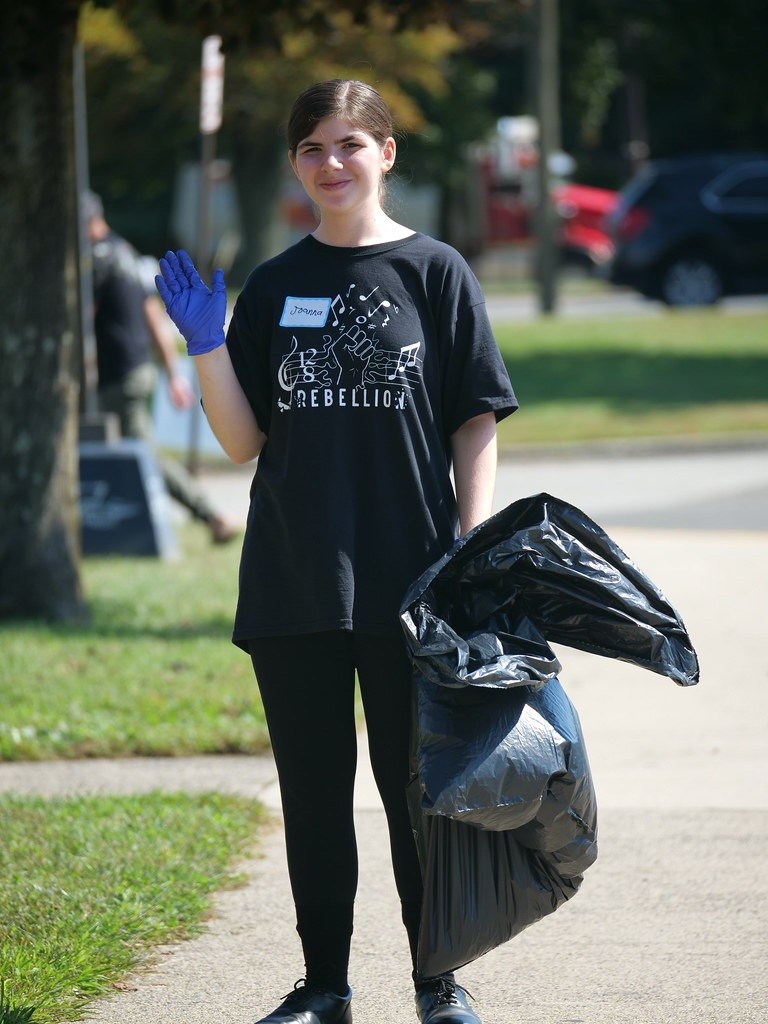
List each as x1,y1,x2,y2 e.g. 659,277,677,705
84,188,243,546
154,79,520,1024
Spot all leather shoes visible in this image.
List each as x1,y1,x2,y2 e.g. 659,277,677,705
254,978,352,1024
412,969,482,1024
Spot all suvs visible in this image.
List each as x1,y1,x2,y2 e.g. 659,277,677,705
601,146,768,308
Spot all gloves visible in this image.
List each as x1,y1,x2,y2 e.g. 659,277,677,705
155,250,227,356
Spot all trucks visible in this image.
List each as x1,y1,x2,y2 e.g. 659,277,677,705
172,132,631,286
